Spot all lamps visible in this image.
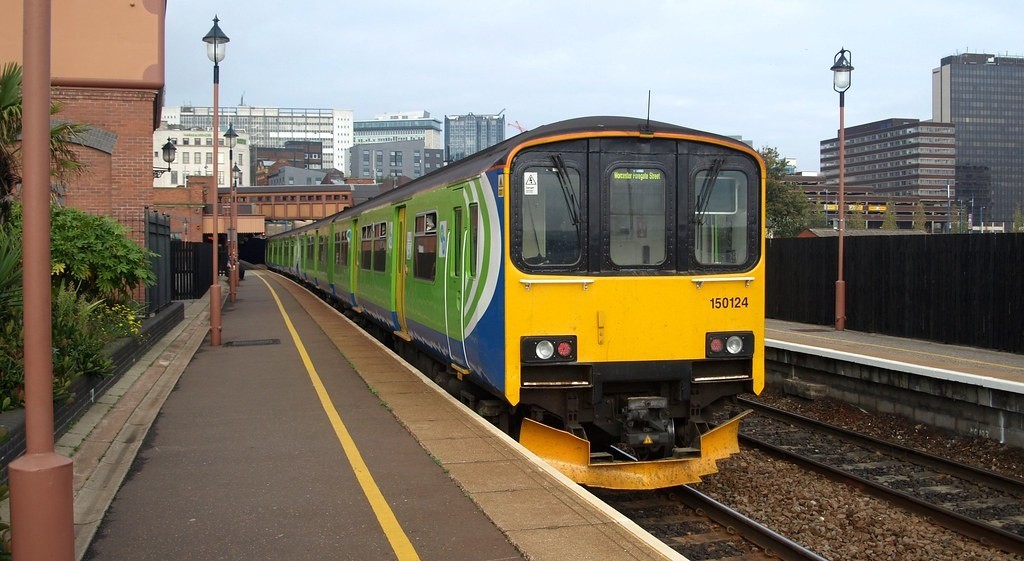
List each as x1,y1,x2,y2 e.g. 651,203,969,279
153,136,178,180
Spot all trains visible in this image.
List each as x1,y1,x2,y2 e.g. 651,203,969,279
262,114,770,495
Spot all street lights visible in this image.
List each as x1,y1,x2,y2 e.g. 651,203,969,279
200,12,231,347
829,45,855,331
222,121,242,304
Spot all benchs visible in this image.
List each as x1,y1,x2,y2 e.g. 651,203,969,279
224,267,241,281
